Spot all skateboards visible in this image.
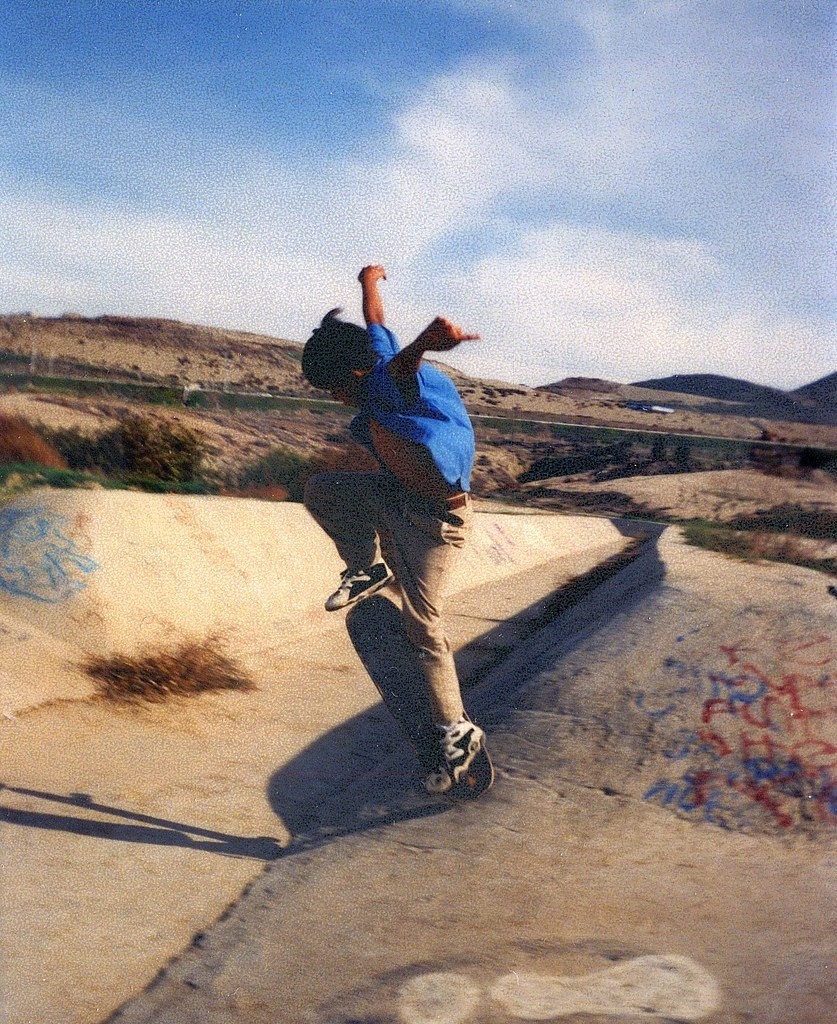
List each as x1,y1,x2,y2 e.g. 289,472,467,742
347,596,495,802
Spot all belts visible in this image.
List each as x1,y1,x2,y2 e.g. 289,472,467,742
398,490,467,527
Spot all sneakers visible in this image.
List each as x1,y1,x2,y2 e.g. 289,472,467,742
324,558,395,612
424,718,487,795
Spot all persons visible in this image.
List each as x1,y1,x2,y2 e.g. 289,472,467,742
302,263,495,793
